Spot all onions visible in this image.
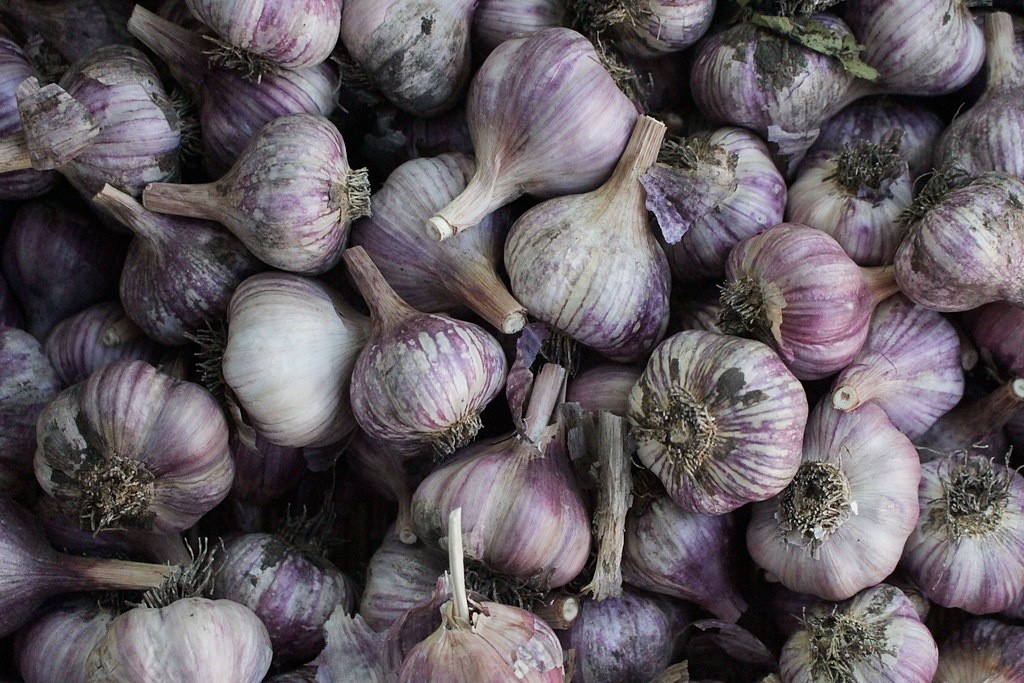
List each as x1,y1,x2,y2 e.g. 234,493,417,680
1,0,1024,683
